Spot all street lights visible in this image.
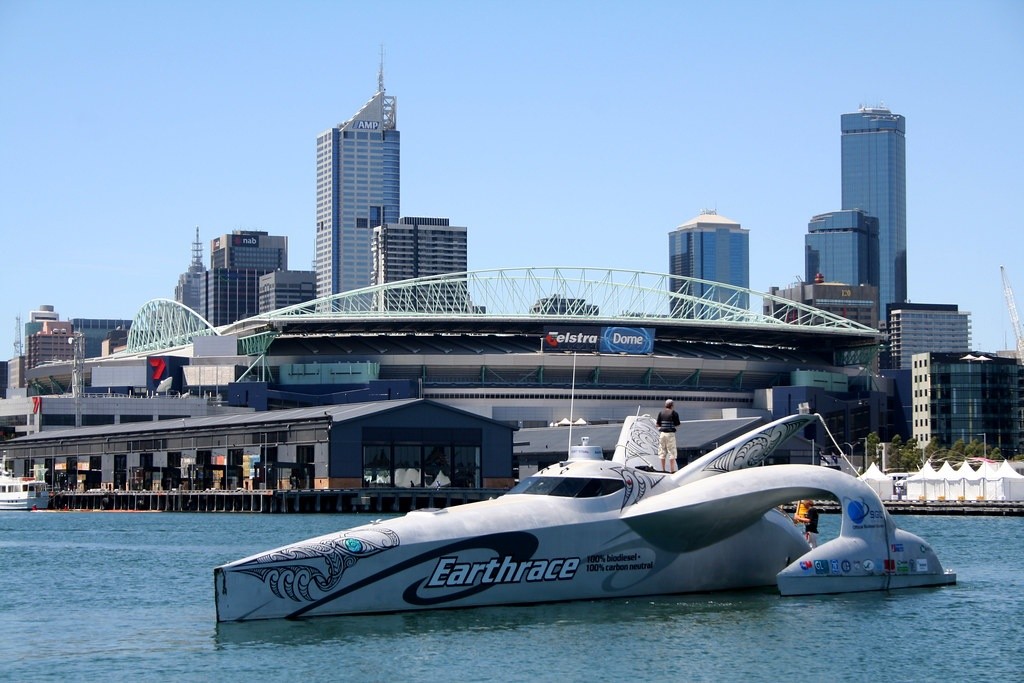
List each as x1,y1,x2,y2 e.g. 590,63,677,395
976,433,987,459
843,442,859,475
860,437,867,473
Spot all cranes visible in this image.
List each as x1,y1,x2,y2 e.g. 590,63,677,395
1001,263,1023,367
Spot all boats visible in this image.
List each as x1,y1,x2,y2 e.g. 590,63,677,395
212,353,957,623
0,450,50,511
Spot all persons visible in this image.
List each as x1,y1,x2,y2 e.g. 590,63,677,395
656,399,680,473
794,500,819,548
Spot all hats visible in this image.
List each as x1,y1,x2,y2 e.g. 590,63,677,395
665,399,673,406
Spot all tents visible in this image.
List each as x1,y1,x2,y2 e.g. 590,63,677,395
857,459,1024,501
